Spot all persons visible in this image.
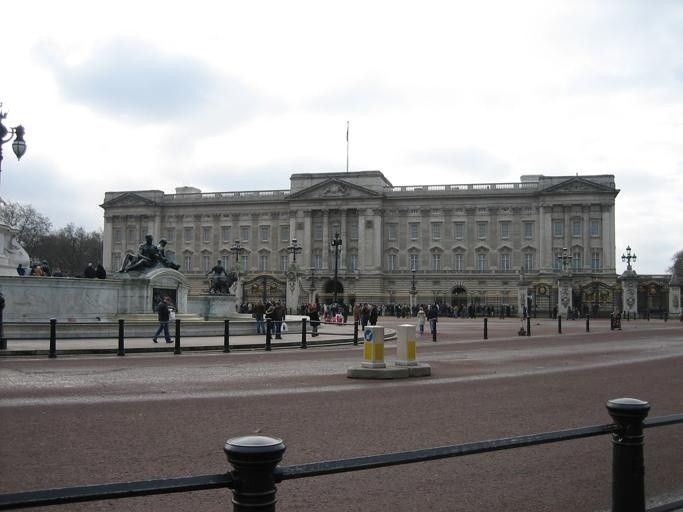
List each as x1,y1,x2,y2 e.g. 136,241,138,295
206,259,227,293
157,293,173,341
17,258,107,278
553,304,557,320
239,300,287,340
583,302,599,318
115,233,161,273
448,304,514,320
152,297,175,343
157,236,180,270
412,303,439,333
521,303,527,320
394,303,411,319
566,305,580,320
352,303,384,326
301,303,348,337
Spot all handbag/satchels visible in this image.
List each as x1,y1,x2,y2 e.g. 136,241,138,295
169,309,176,323
280,323,289,332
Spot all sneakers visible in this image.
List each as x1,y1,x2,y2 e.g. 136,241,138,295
152,339,158,343
166,340,174,343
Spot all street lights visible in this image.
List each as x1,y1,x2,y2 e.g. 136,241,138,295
330,234,343,302
411,269,416,290
287,239,302,262
621,246,637,270
557,248,573,277
310,267,316,290
230,240,244,261
0,101,27,185
